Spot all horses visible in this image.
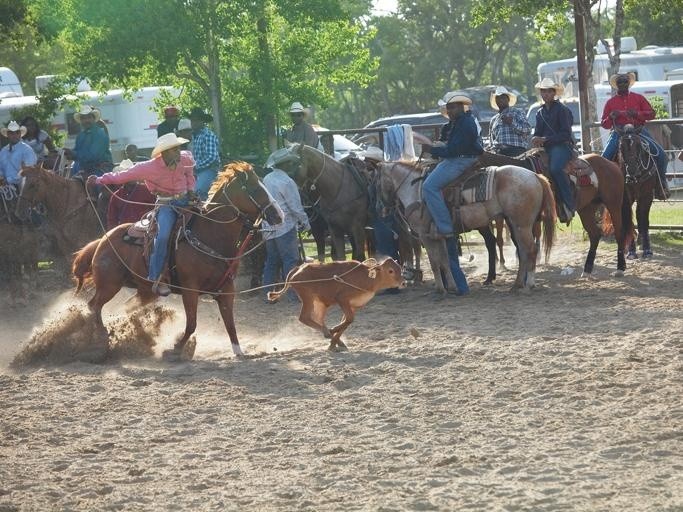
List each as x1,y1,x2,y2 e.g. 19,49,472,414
16,160,120,271
0,180,51,298
70,160,285,358
272,122,669,296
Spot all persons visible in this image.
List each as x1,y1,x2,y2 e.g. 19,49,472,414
598,70,670,200
0,101,219,232
531,77,578,223
254,147,312,304
357,143,400,295
87,132,197,296
419,90,483,240
487,86,532,159
281,101,319,154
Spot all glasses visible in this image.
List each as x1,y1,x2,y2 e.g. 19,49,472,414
617,79,628,84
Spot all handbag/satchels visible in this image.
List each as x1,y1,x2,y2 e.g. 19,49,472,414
567,144,578,160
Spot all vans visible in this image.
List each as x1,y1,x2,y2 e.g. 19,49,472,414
524,98,591,151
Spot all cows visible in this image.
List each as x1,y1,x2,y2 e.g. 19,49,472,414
266,257,407,350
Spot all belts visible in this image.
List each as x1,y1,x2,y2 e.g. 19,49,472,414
157,190,188,201
456,154,476,158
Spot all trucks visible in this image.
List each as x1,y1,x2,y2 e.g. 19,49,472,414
536,35,682,86
0,66,196,166
593,81,682,196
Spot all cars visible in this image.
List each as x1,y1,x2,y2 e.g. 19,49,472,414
279,125,369,165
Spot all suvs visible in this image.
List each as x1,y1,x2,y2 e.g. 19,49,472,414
352,113,455,154
437,86,530,134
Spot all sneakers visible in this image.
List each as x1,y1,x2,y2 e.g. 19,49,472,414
658,186,671,199
152,280,172,296
424,226,454,238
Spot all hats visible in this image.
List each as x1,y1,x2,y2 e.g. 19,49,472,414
112,158,137,172
0,120,27,138
74,105,100,123
151,132,190,158
438,78,564,121
362,147,383,162
184,107,214,123
609,71,636,90
266,148,300,169
286,101,310,122
